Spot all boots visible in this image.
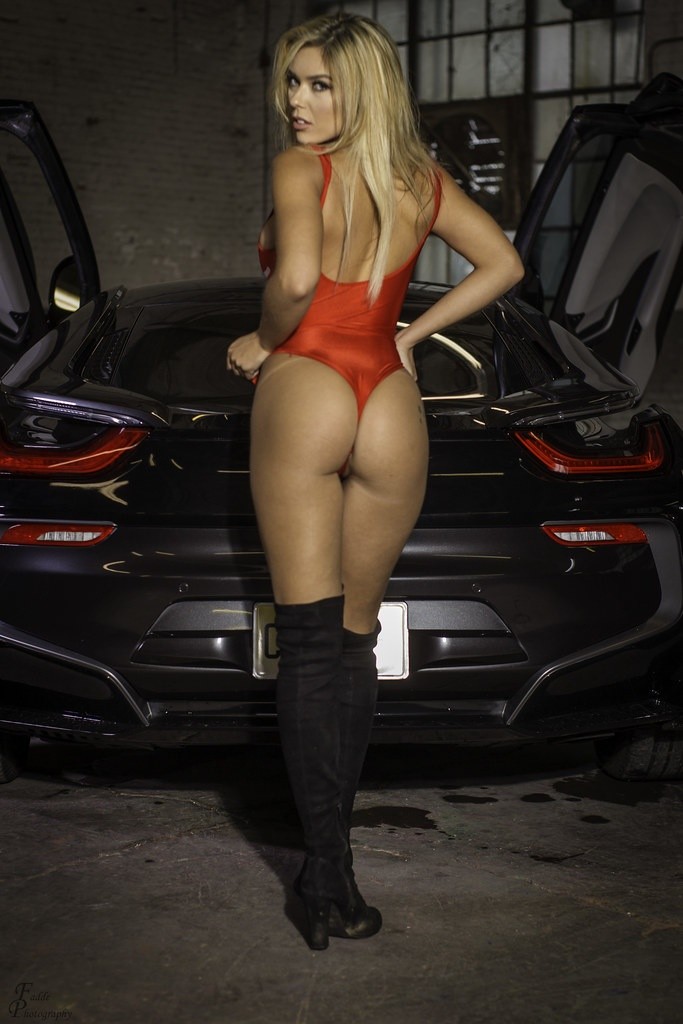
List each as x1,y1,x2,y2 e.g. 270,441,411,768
271,595,383,953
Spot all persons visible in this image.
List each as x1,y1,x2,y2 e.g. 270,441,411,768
230,12,524,950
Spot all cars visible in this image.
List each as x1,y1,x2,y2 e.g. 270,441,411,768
0,73,683,780
